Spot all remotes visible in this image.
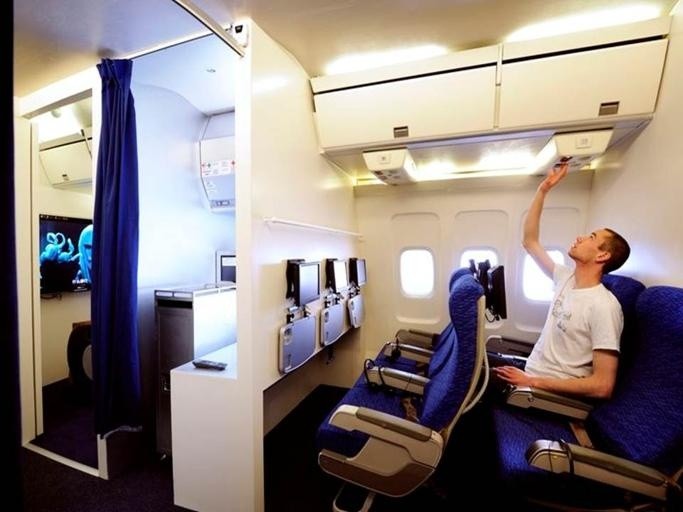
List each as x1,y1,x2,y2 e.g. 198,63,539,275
192,359,227,370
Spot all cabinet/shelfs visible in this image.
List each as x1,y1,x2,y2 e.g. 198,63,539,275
151,280,239,462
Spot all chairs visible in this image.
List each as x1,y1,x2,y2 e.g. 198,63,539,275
316,257,682,512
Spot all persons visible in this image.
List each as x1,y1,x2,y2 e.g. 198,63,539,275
75,225,94,283
477,161,630,424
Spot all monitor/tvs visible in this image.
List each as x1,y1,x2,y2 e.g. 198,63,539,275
290,259,320,307
470,258,507,319
215,251,236,287
39,214,94,294
352,259,367,287
329,259,349,294
